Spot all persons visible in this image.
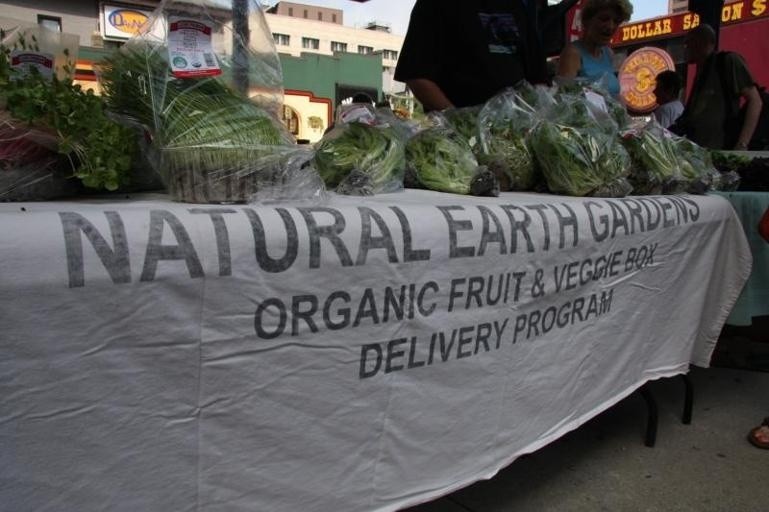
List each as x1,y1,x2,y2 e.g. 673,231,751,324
652,70,685,130
392,1,553,119
322,92,373,136
546,1,633,96
675,22,763,152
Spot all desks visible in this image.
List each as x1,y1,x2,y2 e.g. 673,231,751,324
685,190,768,424
3,191,751,507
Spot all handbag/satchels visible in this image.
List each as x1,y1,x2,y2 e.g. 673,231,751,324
715,50,769,151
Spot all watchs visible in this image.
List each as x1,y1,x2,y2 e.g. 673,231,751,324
737,140,749,149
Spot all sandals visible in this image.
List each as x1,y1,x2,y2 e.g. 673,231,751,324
746,415,769,450
